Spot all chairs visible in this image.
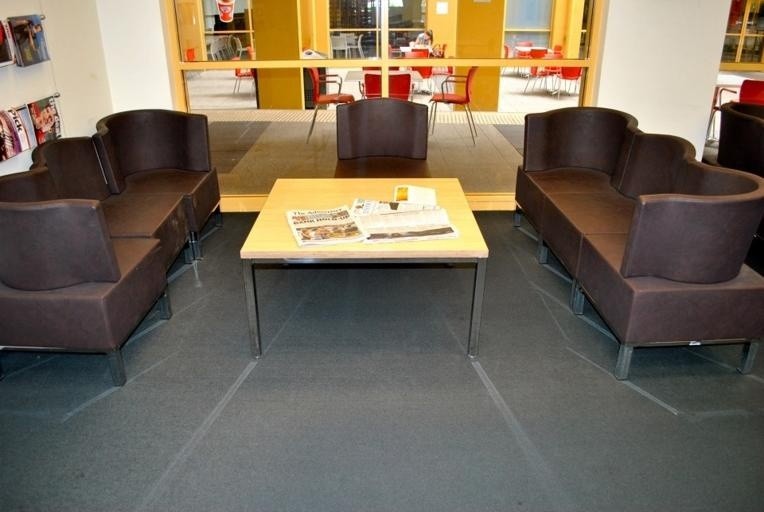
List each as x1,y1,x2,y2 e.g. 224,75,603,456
299,33,478,145
333,97,431,178
184,34,256,95
504,40,583,100
706,79,764,141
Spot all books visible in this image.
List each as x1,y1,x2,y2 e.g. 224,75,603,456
0,12,64,163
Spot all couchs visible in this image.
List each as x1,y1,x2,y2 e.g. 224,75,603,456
515,106,764,381
0,109,222,387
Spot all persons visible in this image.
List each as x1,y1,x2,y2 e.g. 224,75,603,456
300,222,358,241
414,29,434,47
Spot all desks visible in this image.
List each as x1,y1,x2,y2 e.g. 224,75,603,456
705,74,754,141
